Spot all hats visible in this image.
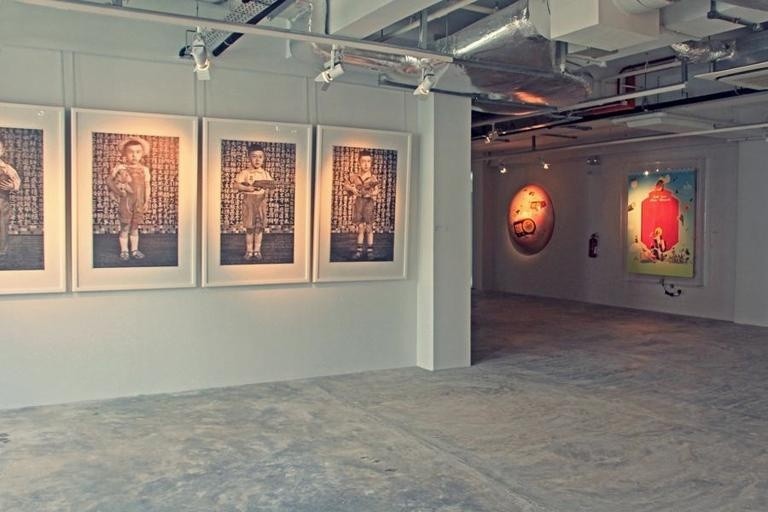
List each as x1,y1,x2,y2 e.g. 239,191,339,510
116,135,152,159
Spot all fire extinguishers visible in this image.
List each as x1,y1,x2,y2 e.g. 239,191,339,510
589,231,599,258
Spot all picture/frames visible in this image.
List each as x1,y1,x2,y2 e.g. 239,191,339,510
70,107,200,295
0,99,71,296
201,114,313,294
312,124,414,288
621,158,709,289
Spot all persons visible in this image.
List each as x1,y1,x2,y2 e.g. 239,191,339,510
342,151,382,258
0,136,21,257
104,136,153,259
234,145,273,261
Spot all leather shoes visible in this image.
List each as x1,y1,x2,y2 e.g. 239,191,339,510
118,250,130,263
242,250,254,260
365,245,376,261
130,249,145,260
352,245,363,262
253,251,263,263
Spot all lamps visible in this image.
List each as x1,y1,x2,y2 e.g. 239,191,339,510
191,25,212,83
412,56,436,97
315,45,345,92
